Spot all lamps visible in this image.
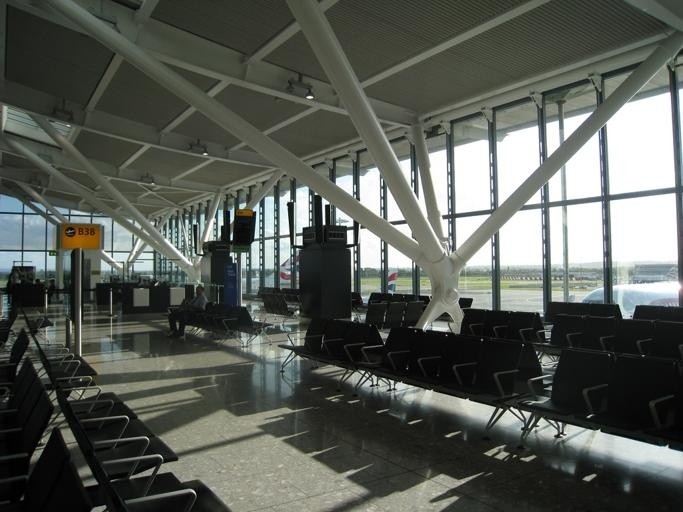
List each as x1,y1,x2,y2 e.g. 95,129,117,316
287,73,314,100
50,99,73,123
139,172,156,186
188,138,209,157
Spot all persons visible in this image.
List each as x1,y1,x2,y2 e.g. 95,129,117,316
6,268,55,305
168,286,207,340
